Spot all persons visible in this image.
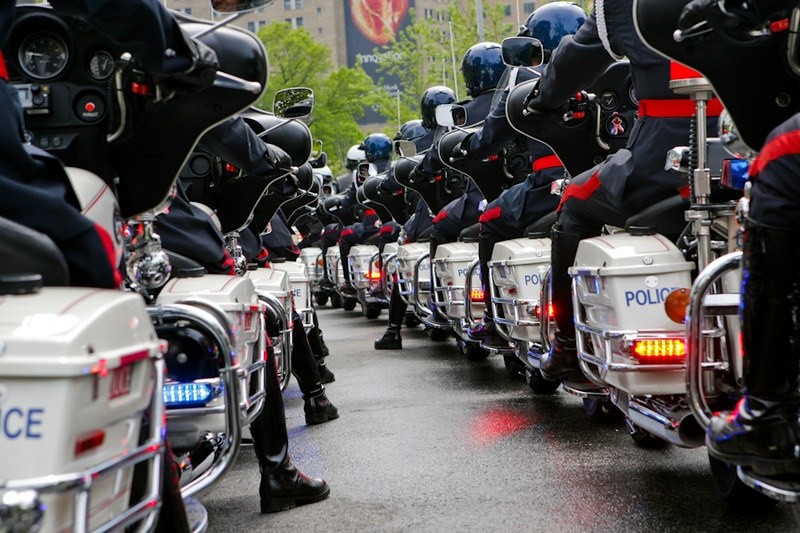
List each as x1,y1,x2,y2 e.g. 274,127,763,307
128,0,799,532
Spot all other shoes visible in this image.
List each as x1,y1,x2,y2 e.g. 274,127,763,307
341,284,355,293
371,289,390,298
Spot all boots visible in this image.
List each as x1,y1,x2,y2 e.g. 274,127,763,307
128,422,192,533
467,237,510,345
428,235,448,324
269,318,338,423
249,345,331,513
705,217,800,476
375,283,407,349
538,223,600,382
308,324,334,383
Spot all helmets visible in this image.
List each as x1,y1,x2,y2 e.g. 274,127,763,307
311,165,333,180
358,133,393,162
393,120,431,142
513,2,587,48
460,42,506,98
421,86,458,128
346,145,367,160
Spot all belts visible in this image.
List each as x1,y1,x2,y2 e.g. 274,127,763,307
531,155,562,171
638,97,724,118
364,209,376,216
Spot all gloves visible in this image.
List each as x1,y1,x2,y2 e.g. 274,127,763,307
186,35,220,104
450,132,476,173
679,0,723,39
523,88,563,122
263,142,291,180
409,164,425,184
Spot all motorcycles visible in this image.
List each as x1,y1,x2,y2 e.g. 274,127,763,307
1,1,800,533
